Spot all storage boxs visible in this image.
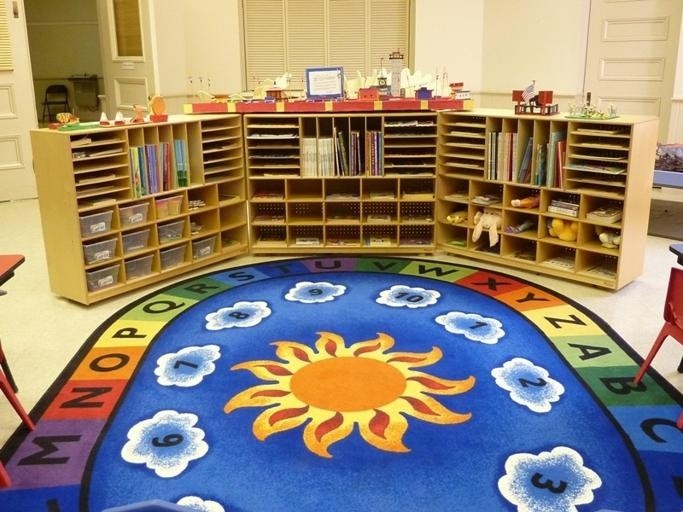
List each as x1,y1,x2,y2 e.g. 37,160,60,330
79,195,218,291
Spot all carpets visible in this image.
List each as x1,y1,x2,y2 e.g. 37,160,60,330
0,253,682,510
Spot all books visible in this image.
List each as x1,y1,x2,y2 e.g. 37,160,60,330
70,133,188,214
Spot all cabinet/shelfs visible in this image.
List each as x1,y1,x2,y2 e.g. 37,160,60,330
240,113,436,253
436,110,661,292
28,112,252,305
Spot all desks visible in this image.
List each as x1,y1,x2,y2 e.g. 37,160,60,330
0,253,38,488
667,243,681,264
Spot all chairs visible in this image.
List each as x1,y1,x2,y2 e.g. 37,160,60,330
633,266,682,435
40,85,71,124
